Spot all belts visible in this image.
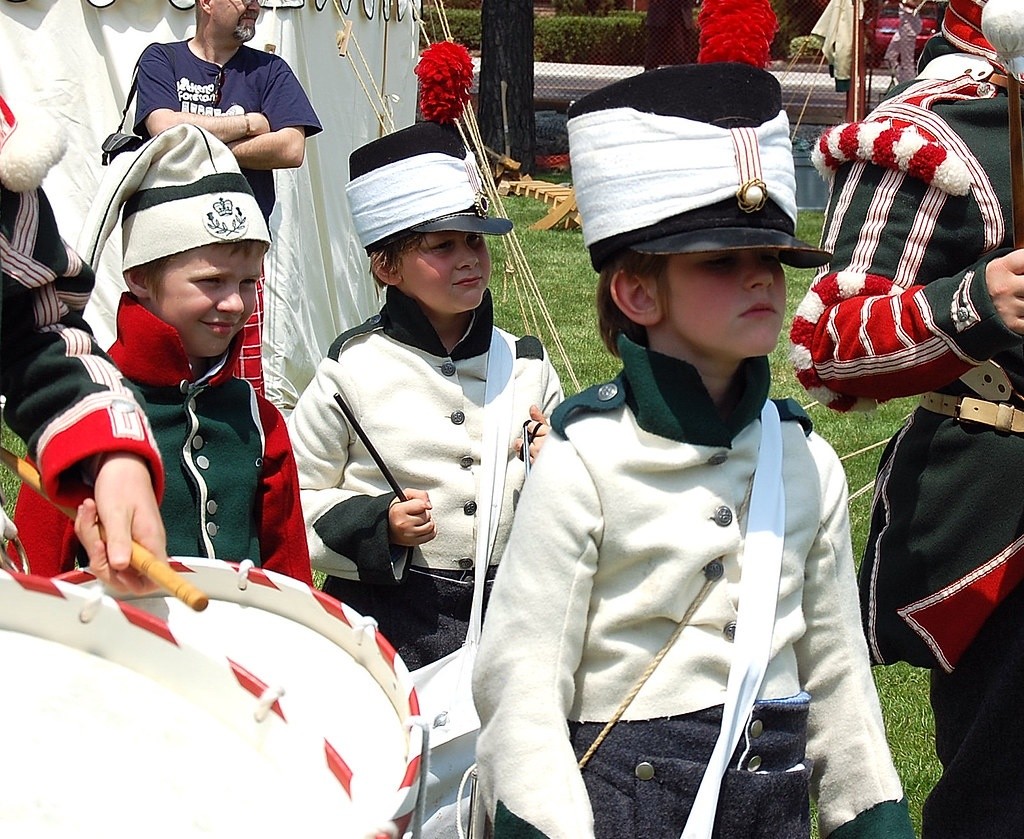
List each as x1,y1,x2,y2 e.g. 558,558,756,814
919,391,1024,433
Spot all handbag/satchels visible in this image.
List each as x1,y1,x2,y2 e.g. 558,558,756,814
102,132,143,166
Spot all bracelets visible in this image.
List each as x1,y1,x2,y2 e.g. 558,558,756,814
243,114,252,135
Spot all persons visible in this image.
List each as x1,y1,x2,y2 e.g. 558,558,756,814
284,120,567,674
0,120,313,594
466,64,917,839
0,98,169,597
787,0,1024,839
132,0,326,396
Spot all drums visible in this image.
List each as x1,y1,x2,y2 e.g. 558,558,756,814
0,564,397,839
42,548,432,839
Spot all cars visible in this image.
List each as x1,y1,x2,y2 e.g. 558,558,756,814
863,1,937,62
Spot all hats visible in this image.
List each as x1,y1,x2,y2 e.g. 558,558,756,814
345,41,513,257
567,0,833,275
73,123,271,272
